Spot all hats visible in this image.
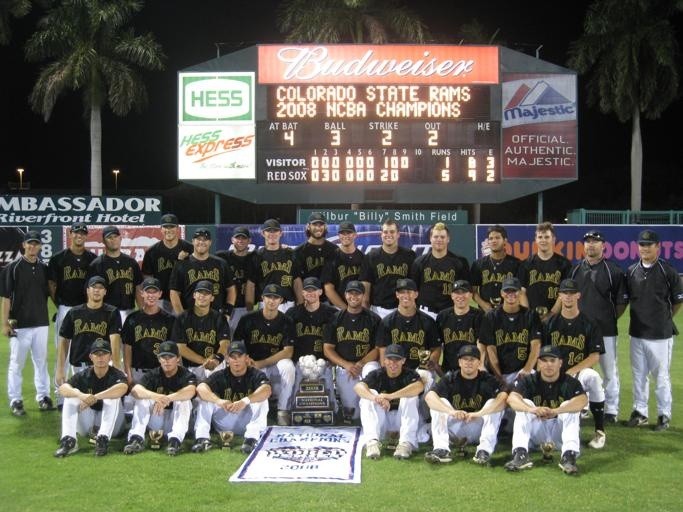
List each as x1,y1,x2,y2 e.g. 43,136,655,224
71,222,120,237
457,345,480,360
339,223,355,233
638,231,659,243
158,341,178,357
396,280,417,291
539,346,561,358
192,228,211,240
262,277,365,296
88,276,215,295
227,341,247,356
502,279,522,291
233,213,325,237
583,231,605,242
558,280,579,291
160,214,178,226
452,280,472,292
385,345,404,360
90,340,112,355
23,229,44,243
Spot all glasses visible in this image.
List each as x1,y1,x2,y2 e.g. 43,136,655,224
584,233,600,238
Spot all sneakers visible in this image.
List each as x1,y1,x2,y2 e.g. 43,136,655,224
123,435,145,454
191,438,213,452
241,438,257,453
394,441,413,459
579,409,669,431
54,436,79,456
39,398,63,410
89,433,109,456
472,450,490,464
167,438,181,455
366,441,381,459
425,448,453,462
505,449,533,470
588,431,606,450
277,410,291,426
10,401,25,418
558,450,578,473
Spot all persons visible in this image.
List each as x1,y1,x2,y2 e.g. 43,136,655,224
1,214,682,476
